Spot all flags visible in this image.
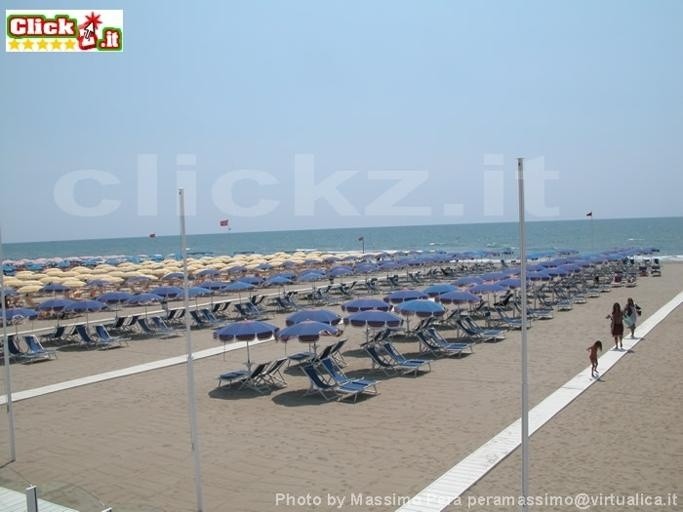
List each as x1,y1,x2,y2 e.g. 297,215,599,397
219,220,229,226
586,212,592,216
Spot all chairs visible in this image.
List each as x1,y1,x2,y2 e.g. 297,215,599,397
0,253,661,406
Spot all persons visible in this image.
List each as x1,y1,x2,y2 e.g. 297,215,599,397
586,340,602,377
